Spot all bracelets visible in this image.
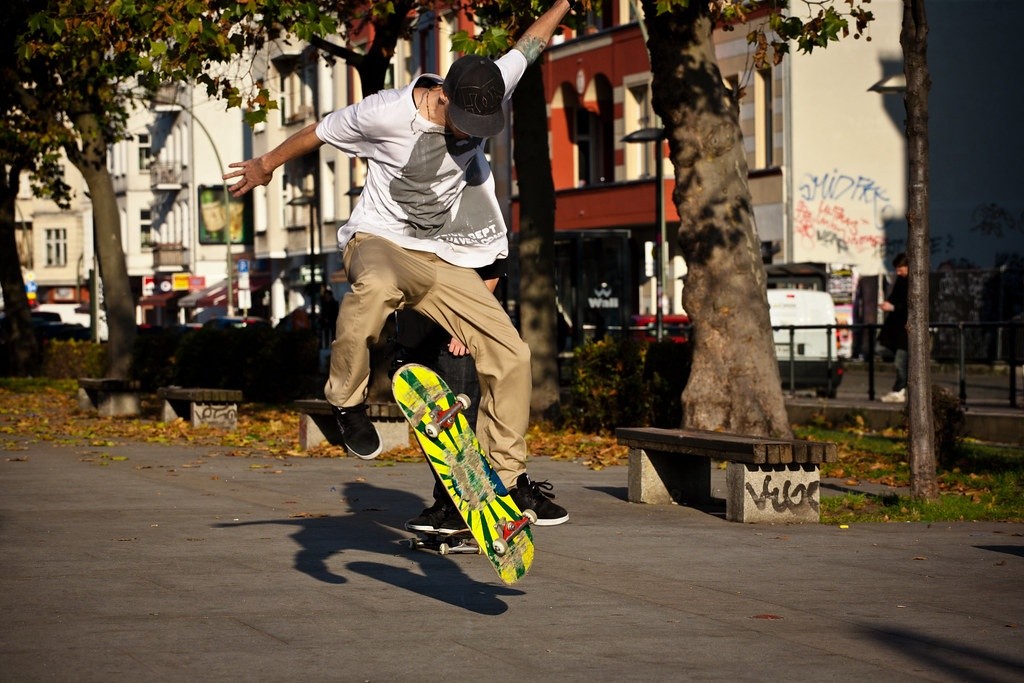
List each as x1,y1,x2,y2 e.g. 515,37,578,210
567,1,577,9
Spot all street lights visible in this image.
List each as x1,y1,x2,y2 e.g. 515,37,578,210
288,196,317,340
620,126,673,343
147,93,234,317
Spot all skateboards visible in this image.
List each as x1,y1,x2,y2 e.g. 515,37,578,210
407,528,483,555
391,362,538,585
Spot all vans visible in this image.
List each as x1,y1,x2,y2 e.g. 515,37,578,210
766,287,846,398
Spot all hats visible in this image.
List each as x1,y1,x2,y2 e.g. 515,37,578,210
442,55,505,138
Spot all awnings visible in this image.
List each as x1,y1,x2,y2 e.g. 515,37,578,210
178,276,269,308
143,293,178,306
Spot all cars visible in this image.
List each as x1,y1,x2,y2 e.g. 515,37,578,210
32,297,107,343
183,304,273,334
629,312,690,348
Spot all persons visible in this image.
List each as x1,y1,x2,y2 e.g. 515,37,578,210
224,0,579,533
877,252,909,402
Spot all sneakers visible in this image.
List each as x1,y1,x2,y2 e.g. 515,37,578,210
509,473,569,525
407,498,470,534
331,400,383,460
